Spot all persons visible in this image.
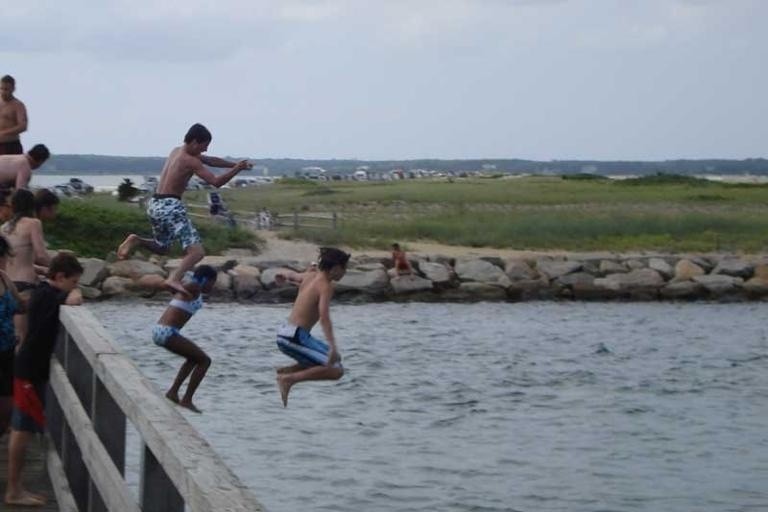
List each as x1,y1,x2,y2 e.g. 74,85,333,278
1,74,84,507
391,243,416,282
116,123,254,298
132,265,219,414
273,247,351,410
206,190,223,215
259,208,272,226
306,264,317,272
